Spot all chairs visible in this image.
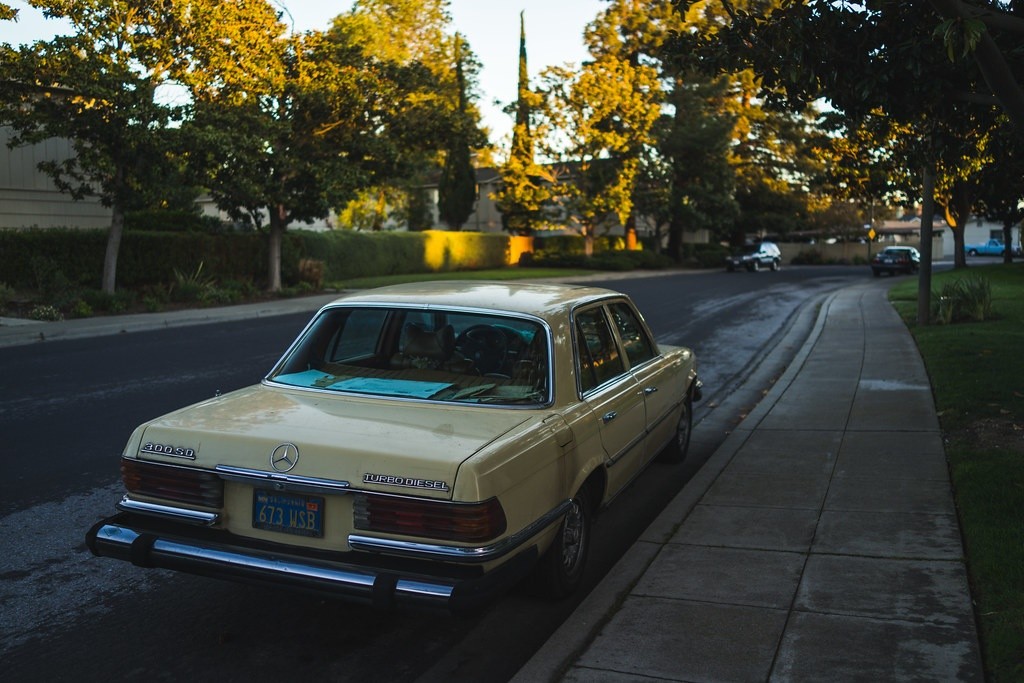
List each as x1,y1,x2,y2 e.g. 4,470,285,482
512,329,590,387
390,325,471,376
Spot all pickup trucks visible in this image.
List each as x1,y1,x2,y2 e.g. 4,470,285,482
965,239,1022,259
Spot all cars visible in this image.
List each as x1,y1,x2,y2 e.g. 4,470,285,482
85,279,703,609
871,245,921,277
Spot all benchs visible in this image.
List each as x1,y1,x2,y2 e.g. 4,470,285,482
322,360,532,404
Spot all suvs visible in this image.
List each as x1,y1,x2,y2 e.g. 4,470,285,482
727,240,782,273
